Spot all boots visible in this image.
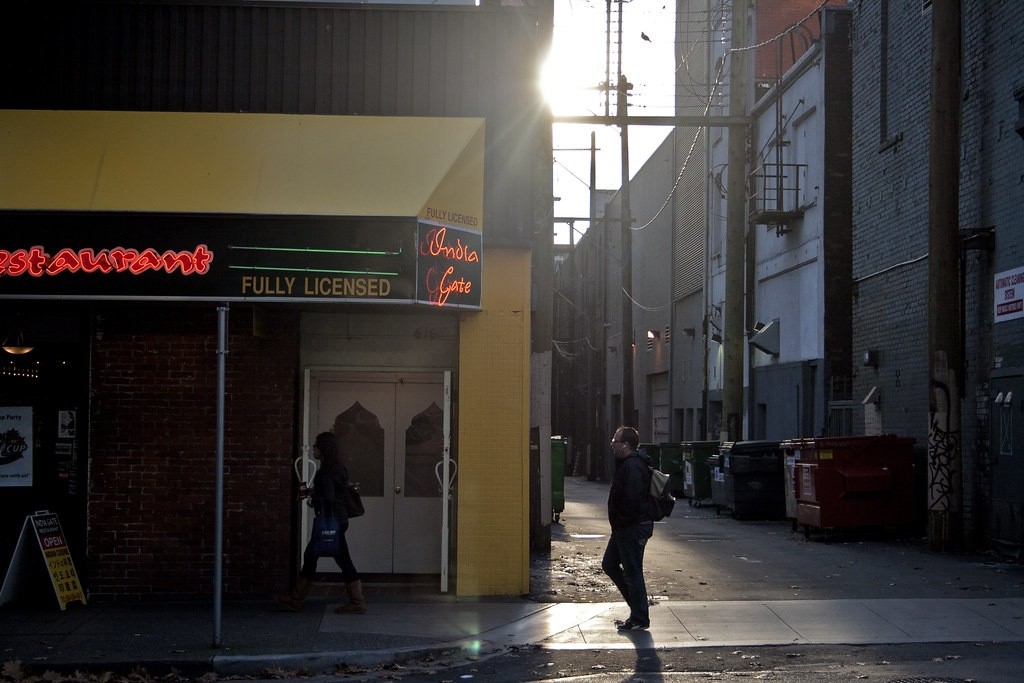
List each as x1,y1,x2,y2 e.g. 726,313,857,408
274,576,312,613
334,579,367,615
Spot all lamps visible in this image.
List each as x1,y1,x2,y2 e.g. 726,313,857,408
607,346,617,353
0,299,34,354
647,329,657,340
683,328,695,336
95,309,106,340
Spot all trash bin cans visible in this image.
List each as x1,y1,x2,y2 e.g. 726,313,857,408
681,441,720,508
636,443,658,468
551,433,568,523
704,454,724,515
778,433,917,542
718,440,784,520
657,442,683,498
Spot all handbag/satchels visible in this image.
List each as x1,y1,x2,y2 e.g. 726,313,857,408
334,479,365,518
311,498,340,558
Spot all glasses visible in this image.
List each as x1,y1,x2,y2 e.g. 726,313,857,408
312,444,317,450
612,439,626,444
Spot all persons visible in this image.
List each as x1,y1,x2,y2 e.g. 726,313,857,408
601,425,654,631
275,432,367,614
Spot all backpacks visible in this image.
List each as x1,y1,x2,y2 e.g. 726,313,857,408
648,466,676,522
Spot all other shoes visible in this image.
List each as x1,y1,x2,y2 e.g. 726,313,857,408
617,618,649,631
